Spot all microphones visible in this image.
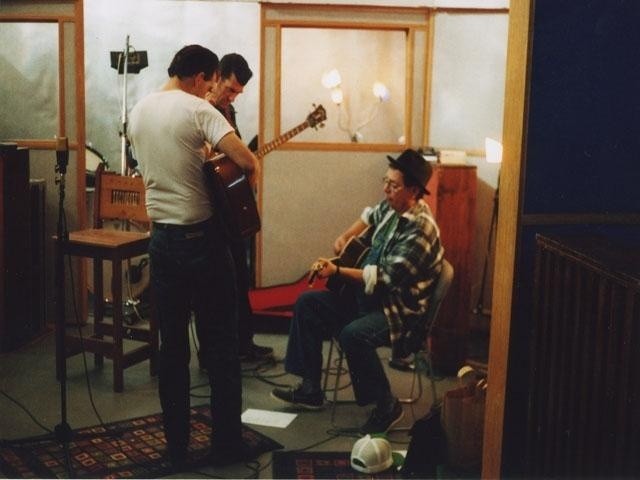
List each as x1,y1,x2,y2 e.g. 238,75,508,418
55,136,69,174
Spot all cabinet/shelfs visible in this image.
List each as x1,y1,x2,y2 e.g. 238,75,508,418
423,159,478,378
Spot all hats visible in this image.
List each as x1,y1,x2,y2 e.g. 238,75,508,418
350,432,405,474
387,148,432,194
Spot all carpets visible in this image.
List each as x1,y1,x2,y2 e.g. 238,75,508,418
271,449,399,480
0,402,285,479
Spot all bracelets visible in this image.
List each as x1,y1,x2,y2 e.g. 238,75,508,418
336,265,340,277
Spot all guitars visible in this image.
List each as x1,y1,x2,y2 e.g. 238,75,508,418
206,150,261,238
306,223,375,290
255,103,328,159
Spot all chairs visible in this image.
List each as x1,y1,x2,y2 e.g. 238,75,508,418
322,257,455,433
50,163,162,392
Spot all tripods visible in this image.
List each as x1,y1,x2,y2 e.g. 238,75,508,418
0,236,123,479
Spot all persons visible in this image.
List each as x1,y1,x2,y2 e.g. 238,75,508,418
124,43,262,468
270,149,446,435
197,51,276,370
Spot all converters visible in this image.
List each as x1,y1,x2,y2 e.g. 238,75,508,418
388,356,409,370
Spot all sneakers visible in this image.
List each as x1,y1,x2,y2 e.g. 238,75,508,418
238,342,274,360
270,382,325,410
357,398,405,437
